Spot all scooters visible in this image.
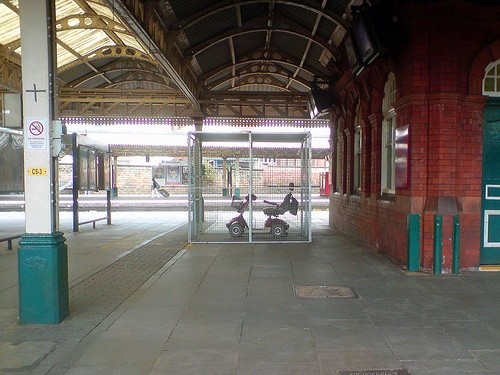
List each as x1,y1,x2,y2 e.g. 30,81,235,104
226,194,290,238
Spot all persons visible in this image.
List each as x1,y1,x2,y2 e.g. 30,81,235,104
151,178,160,199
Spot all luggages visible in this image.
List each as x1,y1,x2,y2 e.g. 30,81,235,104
160,189,169,198
